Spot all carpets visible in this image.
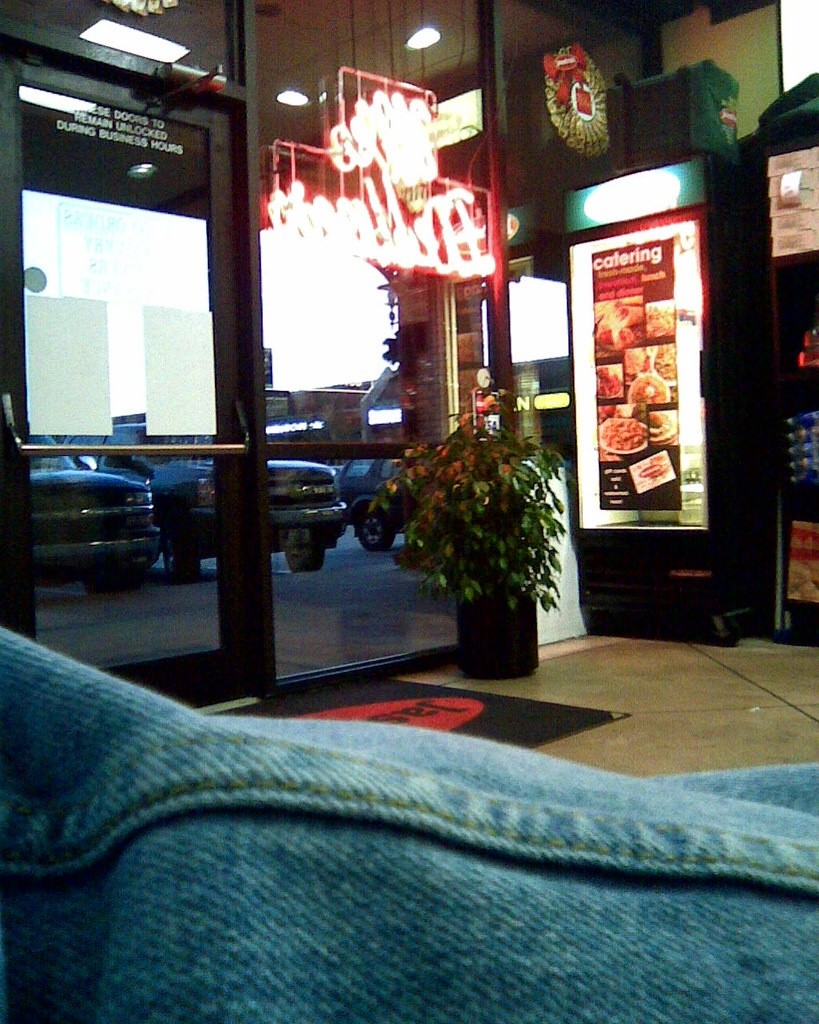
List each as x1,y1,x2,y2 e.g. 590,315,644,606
207,680,631,749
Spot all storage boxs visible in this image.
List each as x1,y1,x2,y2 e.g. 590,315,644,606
605,60,741,175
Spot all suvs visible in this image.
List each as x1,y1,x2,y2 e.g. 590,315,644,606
27,435,160,596
56,422,349,588
337,458,412,552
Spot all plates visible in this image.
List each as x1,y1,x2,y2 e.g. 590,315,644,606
589,295,680,455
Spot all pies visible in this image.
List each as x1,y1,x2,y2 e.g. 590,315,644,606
595,305,646,348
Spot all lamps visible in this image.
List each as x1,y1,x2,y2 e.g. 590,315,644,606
276,88,309,107
404,25,441,50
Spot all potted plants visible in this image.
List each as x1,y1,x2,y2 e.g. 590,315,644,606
368,389,567,678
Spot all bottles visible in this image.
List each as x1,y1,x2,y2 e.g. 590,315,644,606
784,408,819,483
